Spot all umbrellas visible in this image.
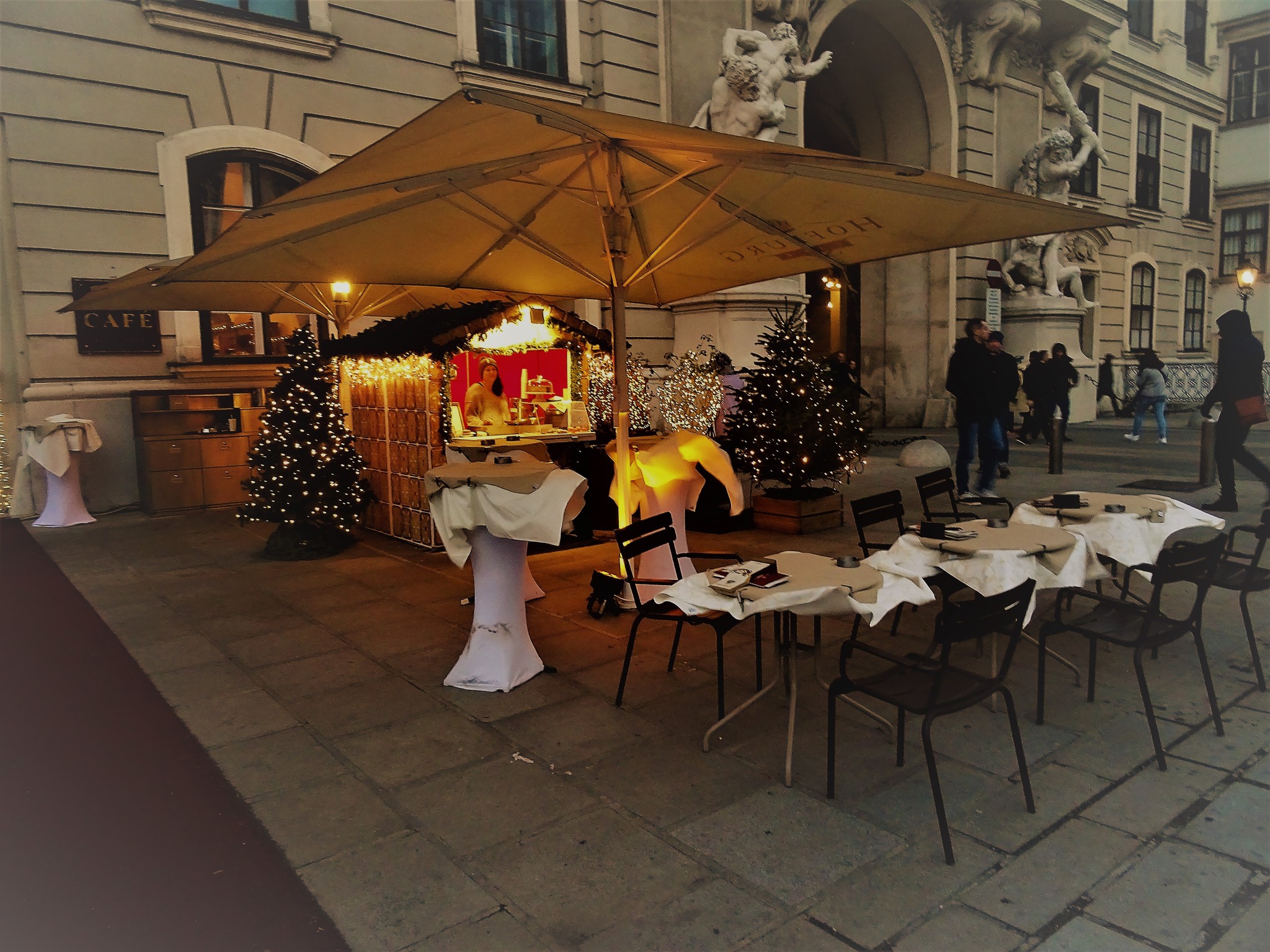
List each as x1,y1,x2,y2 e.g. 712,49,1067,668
149,87,1143,605
53,252,580,340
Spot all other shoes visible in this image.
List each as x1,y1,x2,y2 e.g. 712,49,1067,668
1063,435,1072,441
1156,438,1167,444
1124,433,1137,442
1201,414,1214,423
1016,437,1031,445
1201,496,1238,512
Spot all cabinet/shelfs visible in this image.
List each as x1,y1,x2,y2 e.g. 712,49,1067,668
130,385,277,517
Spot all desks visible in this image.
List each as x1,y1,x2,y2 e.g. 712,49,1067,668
446,430,596,555
859,517,1119,715
652,549,936,791
1006,490,1226,653
423,461,589,695
16,414,103,528
604,428,742,610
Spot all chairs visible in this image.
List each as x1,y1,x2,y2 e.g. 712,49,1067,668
613,467,1270,865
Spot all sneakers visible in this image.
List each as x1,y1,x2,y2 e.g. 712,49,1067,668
980,490,998,497
958,492,983,505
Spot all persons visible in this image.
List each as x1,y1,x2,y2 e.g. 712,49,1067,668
1013,348,1074,447
713,352,747,437
1123,349,1169,443
985,330,1020,478
1096,353,1126,417
1040,343,1080,475
1200,309,1270,512
688,21,833,142
1000,111,1102,308
464,356,519,426
944,318,1002,505
